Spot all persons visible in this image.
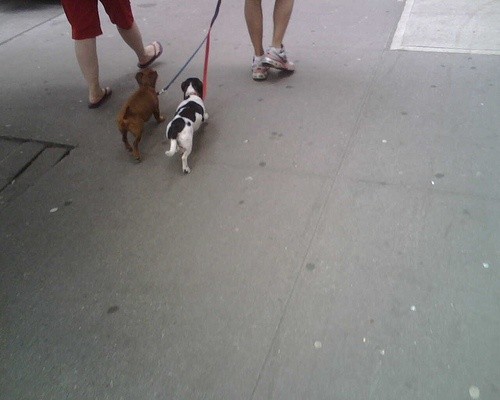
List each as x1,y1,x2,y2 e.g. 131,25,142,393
59,0,162,108
244,0,294,80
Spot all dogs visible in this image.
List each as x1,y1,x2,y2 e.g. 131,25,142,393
118,67,166,163
164,77,210,174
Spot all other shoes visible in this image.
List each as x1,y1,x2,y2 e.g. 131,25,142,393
251,56,270,79
262,44,296,71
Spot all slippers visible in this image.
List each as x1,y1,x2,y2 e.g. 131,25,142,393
88,86,112,109
137,41,162,68
